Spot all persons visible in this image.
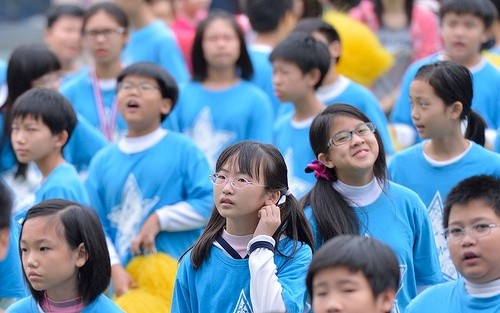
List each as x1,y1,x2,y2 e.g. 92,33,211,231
0,0,500,313
171,140,315,313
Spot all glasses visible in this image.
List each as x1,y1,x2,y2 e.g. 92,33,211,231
327,122,376,147
84,26,125,39
208,172,268,190
115,81,160,94
439,220,500,239
